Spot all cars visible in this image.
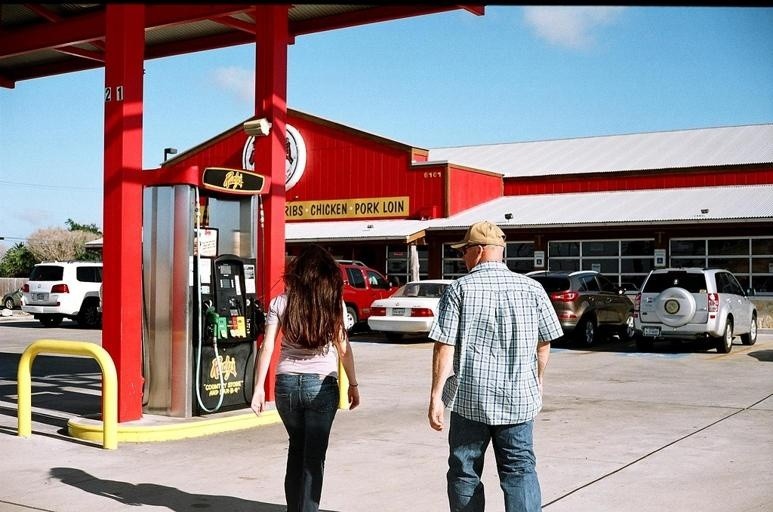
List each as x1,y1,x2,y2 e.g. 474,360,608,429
368,279,457,339
523,270,634,348
2,287,22,310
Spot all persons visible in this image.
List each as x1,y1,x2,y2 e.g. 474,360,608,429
426,221,564,512
250,243,360,512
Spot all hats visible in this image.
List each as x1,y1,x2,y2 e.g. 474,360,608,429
450,220,506,250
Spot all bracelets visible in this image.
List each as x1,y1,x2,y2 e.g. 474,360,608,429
350,384,358,387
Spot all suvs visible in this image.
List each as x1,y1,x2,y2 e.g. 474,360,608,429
633,267,758,353
20,260,103,326
337,260,399,336
